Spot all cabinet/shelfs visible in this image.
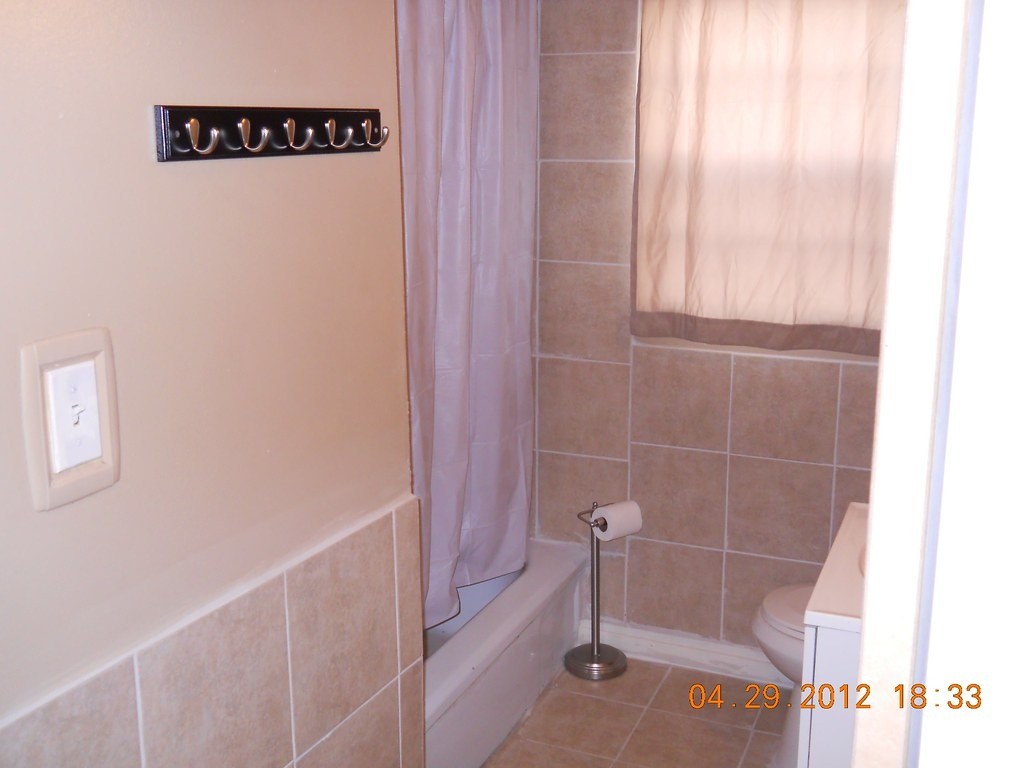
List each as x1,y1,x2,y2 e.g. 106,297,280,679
797,502,869,768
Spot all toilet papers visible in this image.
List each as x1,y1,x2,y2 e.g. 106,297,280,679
590,500,642,542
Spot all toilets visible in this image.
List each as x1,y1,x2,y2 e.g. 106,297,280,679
751,583,815,768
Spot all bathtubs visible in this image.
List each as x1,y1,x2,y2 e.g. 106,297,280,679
419,539,587,768
